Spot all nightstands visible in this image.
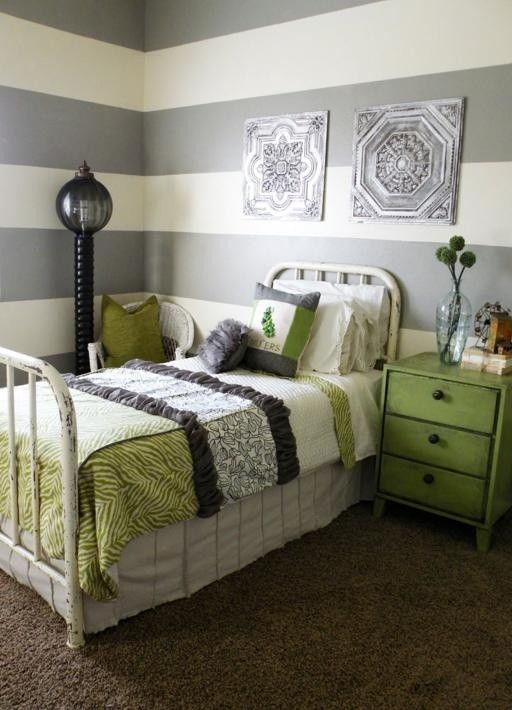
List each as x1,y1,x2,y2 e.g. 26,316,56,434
371,352,511,553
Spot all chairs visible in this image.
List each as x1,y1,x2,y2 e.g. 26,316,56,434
87,300,195,372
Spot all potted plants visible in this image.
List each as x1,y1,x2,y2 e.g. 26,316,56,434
435,235,476,365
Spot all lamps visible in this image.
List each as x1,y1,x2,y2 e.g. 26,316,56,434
55,161,112,375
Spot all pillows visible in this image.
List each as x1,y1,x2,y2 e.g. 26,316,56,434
196,279,390,378
100,292,166,367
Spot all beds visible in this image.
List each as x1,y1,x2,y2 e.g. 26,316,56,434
0,261,402,650
